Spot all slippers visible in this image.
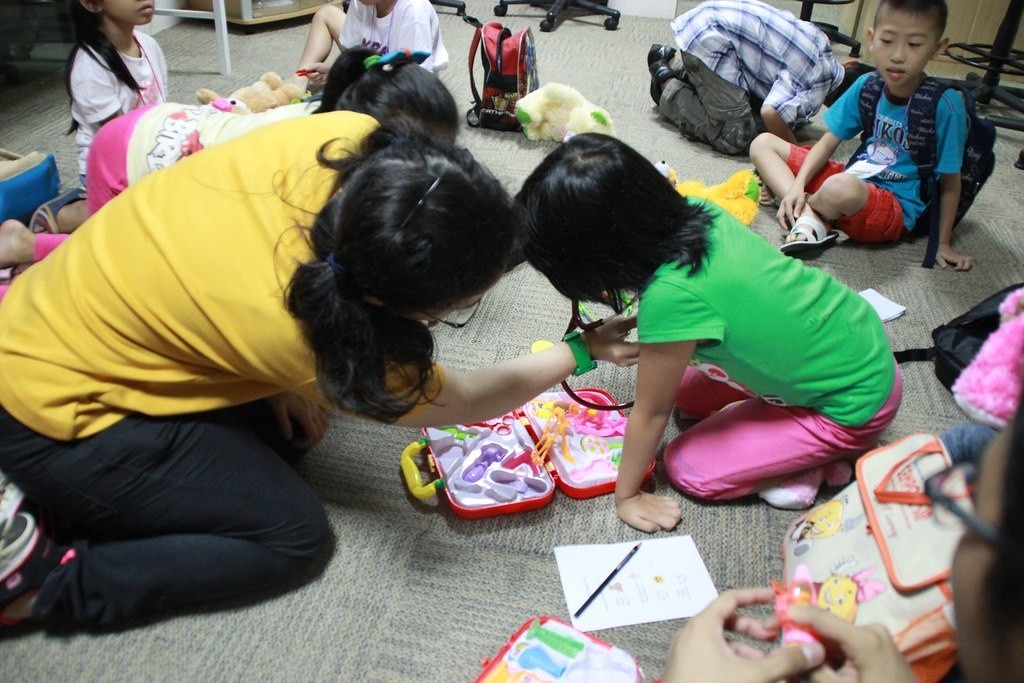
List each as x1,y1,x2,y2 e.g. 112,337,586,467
779,217,839,255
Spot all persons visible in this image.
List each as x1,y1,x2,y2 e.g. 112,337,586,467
31,0,169,232
0,47,460,299
287,0,450,95
660,370,1024,683
750,0,973,271
515,134,905,533
0,111,642,643
648,0,877,156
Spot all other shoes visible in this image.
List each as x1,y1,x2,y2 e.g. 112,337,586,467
0,471,53,609
30,188,87,234
650,59,677,106
648,44,677,67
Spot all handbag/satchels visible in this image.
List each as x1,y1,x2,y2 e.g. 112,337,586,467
933,282,1024,395
772,432,978,683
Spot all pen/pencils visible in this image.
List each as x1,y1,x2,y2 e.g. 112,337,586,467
574,543,642,617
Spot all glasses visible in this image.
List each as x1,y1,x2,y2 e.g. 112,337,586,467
406,301,481,327
923,461,1000,548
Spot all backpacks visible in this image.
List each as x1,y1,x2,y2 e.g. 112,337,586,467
860,70,997,268
463,15,540,132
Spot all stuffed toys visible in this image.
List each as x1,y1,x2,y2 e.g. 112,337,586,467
654,160,762,226
952,288,1024,428
196,73,304,115
516,82,614,145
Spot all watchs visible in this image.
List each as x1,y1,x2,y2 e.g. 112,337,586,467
562,331,598,377
